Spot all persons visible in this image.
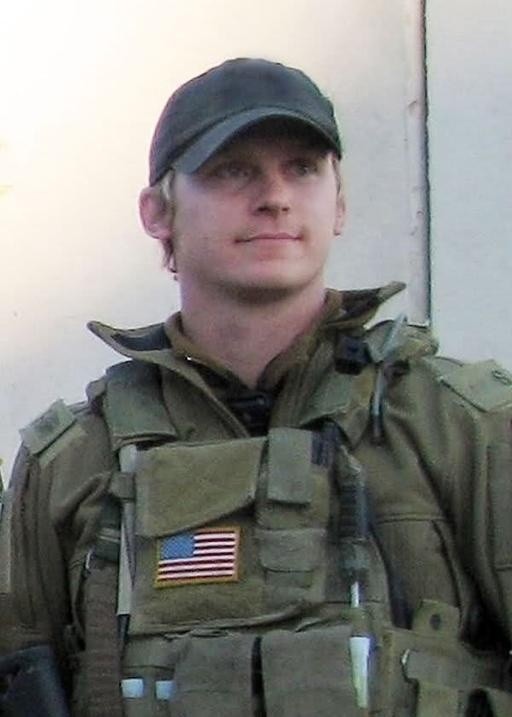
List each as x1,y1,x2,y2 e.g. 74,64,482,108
0,58,512,717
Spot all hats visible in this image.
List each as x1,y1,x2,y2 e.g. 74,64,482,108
148,57,343,187
